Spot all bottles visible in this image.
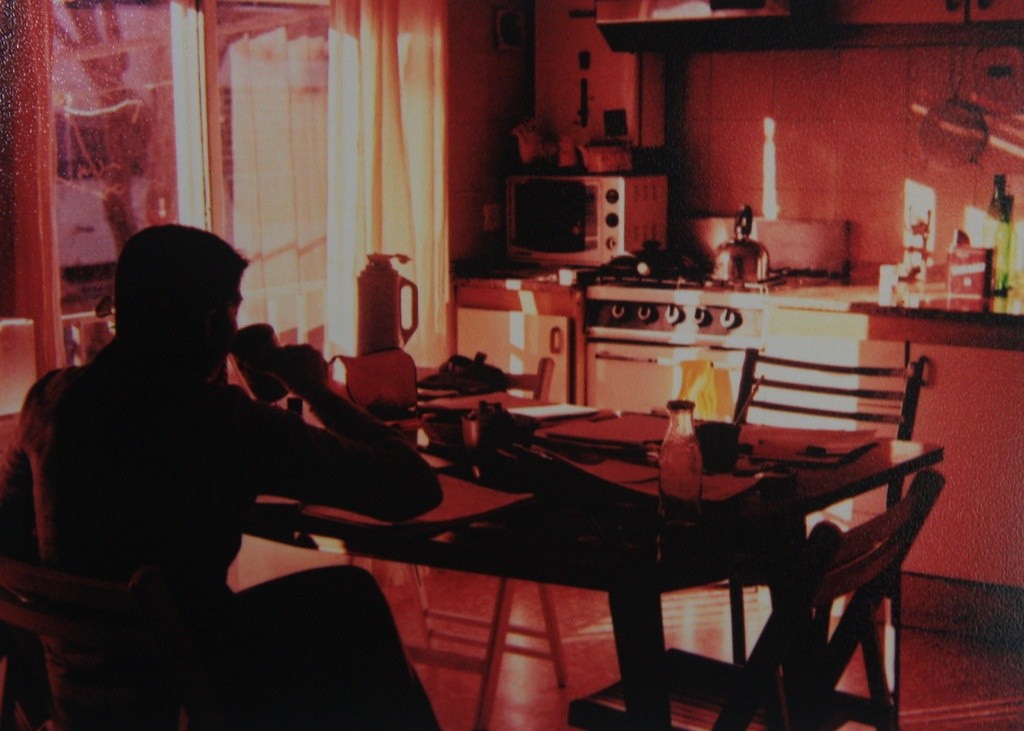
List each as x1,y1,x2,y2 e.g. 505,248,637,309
988,195,1023,315
657,399,704,499
980,173,1006,247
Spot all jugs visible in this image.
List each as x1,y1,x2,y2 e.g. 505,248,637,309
355,252,419,358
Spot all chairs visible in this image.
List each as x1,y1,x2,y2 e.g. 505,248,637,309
346,348,947,731
0,556,227,730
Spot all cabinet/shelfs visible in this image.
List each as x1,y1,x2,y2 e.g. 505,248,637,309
760,340,1024,642
452,285,584,409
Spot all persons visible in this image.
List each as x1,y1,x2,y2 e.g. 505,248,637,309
0,225,443,731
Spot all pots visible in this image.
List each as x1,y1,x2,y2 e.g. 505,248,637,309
576,137,634,172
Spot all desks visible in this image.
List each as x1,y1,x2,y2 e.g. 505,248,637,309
251,389,945,731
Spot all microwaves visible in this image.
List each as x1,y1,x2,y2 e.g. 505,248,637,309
501,174,668,267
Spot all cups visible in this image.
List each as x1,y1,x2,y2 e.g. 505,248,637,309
75,315,112,369
231,322,289,405
695,421,741,475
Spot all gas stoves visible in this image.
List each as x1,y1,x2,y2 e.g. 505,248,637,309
601,239,791,284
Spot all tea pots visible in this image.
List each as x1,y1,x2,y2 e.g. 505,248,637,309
510,117,547,173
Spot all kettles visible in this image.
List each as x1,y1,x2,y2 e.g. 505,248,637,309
708,204,770,283
